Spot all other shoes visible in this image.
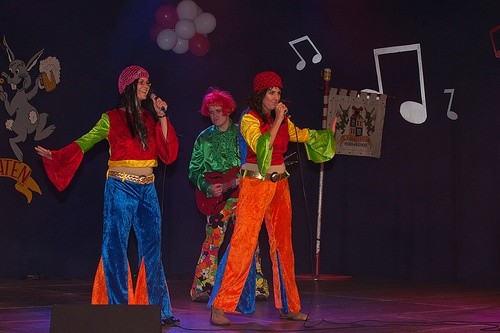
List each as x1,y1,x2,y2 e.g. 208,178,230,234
256,295,266,300
212,307,230,325
279,309,309,321
194,296,209,302
161,316,180,327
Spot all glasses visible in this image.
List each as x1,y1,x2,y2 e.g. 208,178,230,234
268,91,281,95
137,79,151,87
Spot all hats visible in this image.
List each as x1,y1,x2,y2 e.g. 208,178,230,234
118,65,149,94
254,71,283,94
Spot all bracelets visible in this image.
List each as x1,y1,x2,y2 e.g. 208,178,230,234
158,115,166,118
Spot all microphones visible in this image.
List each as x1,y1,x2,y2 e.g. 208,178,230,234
278,101,286,114
150,93,168,112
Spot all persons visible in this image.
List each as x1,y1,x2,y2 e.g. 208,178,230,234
34,67,180,326
207,71,337,324
189,88,271,301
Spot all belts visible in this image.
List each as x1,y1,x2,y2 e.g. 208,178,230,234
107,171,155,185
239,169,290,183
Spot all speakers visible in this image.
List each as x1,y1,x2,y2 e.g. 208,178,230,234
50,303,162,333
